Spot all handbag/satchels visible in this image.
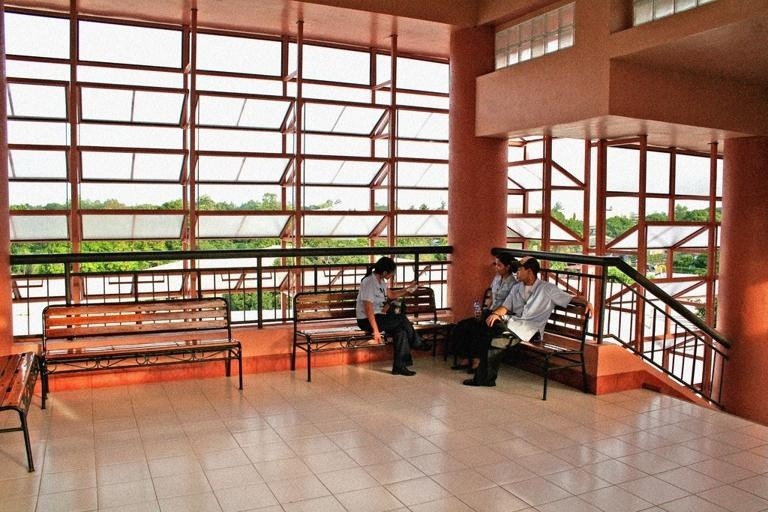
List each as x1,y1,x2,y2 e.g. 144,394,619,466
384,299,407,316
450,317,477,335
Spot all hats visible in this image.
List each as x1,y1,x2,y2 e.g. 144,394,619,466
512,256,540,275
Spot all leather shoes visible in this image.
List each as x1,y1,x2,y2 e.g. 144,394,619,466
451,363,479,386
391,367,416,377
419,343,433,352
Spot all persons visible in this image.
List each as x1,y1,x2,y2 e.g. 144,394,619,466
448,252,520,373
462,256,594,386
355,257,433,376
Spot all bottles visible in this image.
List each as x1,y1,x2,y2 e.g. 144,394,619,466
474,300,483,318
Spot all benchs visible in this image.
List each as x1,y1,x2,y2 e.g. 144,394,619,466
291,286,455,382
38,301,241,394
0,351,51,473
479,288,589,401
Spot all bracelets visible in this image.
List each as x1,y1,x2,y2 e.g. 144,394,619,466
490,311,501,317
